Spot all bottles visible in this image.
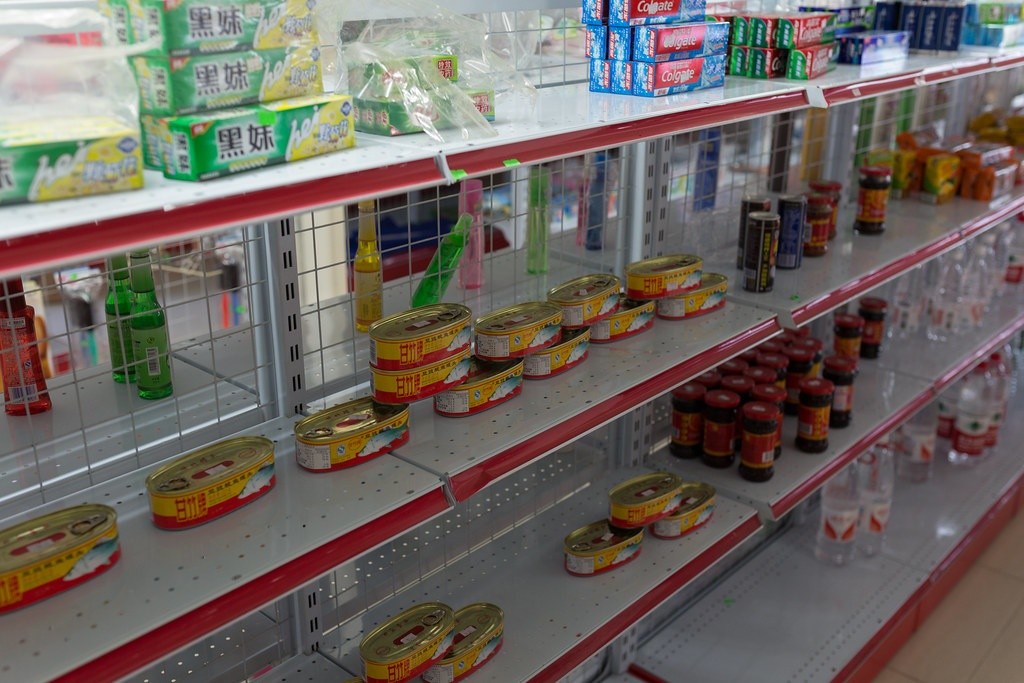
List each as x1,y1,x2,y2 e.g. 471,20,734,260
0,276,52,417
458,180,486,289
354,199,384,333
129,249,174,401
409,212,473,310
670,165,1024,566
105,252,136,384
692,129,721,214
585,151,611,251
527,166,549,274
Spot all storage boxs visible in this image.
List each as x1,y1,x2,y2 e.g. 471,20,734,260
579,0,1024,100
0,0,497,208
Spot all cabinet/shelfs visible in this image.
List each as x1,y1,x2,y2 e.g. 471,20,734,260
0,117,448,683
650,44,1024,583
302,73,939,683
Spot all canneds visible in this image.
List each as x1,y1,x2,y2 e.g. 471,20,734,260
671,297,887,483
0,504,121,616
738,163,893,292
292,255,729,473
339,601,505,683
562,473,716,577
145,435,278,531
